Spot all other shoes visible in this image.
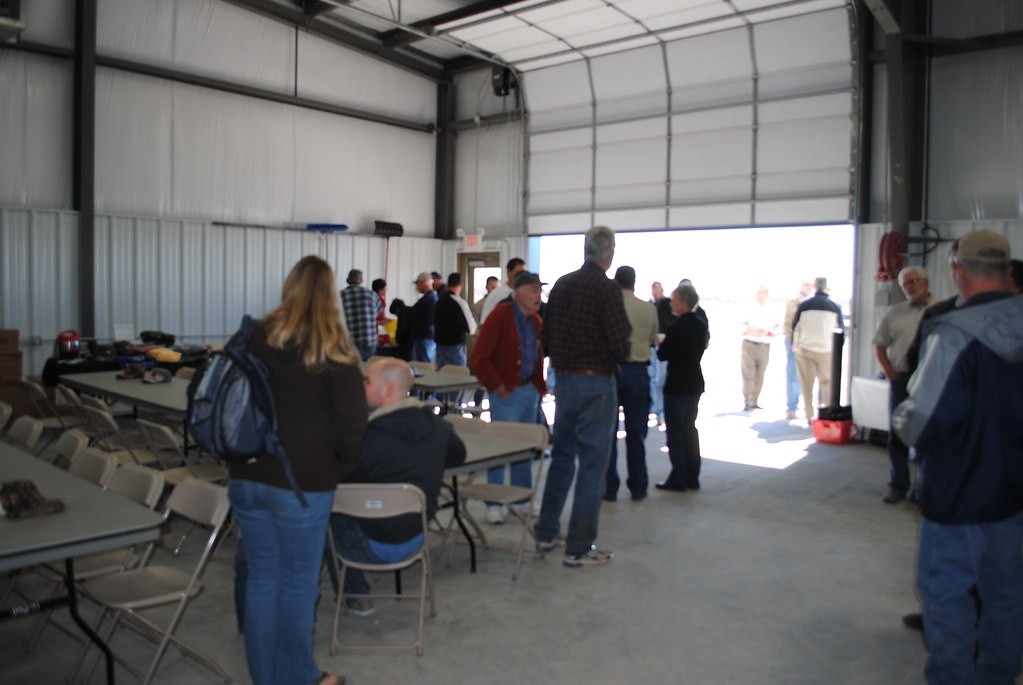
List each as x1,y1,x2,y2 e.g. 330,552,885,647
332,590,374,617
903,610,924,629
314,671,345,684
884,483,907,503
655,478,686,493
603,493,616,502
632,492,646,501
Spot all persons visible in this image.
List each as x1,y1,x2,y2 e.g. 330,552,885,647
469,273,547,525
872,265,939,504
740,285,780,411
543,225,633,568
327,357,467,616
889,229,1023,685
784,277,846,425
342,256,549,391
646,278,711,493
601,265,658,502
222,255,367,685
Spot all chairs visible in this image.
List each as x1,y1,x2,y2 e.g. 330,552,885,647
409,361,470,411
328,482,438,656
0,366,233,684
437,414,549,581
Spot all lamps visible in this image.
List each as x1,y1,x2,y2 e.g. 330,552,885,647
456,228,485,250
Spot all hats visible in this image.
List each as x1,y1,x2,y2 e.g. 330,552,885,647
142,368,170,384
413,273,431,282
117,365,145,379
1,481,66,519
513,272,548,288
957,228,1011,265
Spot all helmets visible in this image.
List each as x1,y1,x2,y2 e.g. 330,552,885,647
56,331,80,359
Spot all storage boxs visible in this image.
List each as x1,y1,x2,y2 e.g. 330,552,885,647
811,418,852,446
0,328,23,379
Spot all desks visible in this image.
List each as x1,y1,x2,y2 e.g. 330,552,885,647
443,434,535,574
851,376,891,443
1,440,167,685
58,369,194,460
362,360,481,412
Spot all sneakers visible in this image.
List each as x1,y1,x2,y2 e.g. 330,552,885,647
538,538,555,552
511,503,543,517
486,505,504,525
563,544,613,566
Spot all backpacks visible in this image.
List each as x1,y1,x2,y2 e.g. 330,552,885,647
180,319,279,465
